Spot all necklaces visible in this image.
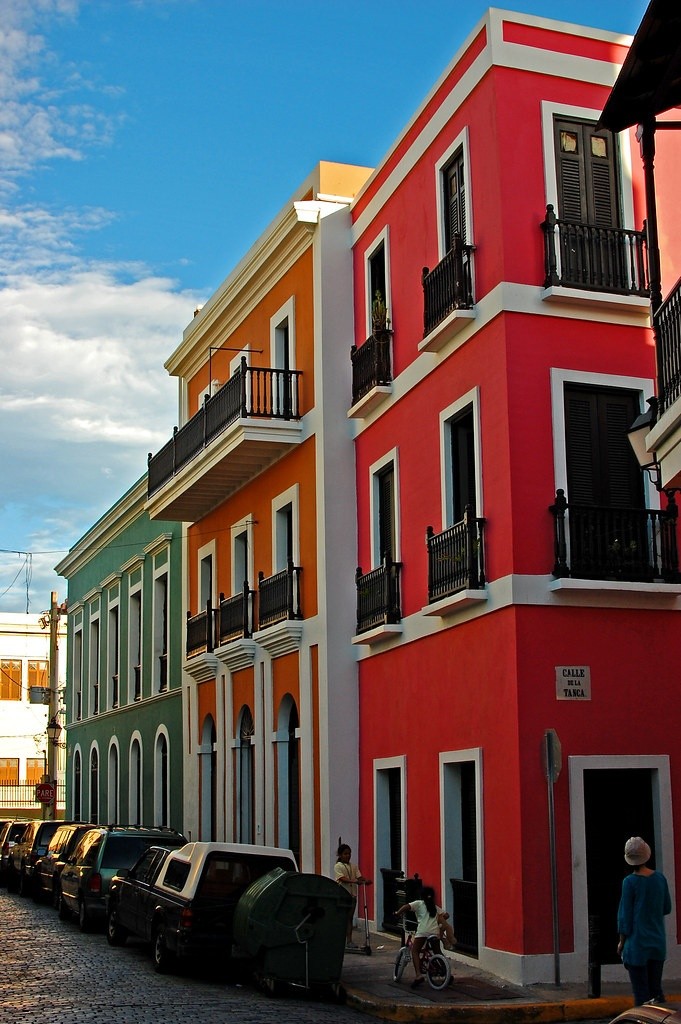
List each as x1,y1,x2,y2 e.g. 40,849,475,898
342,862,352,886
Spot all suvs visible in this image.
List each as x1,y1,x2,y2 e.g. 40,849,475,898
0,817,36,890
32,823,105,912
58,828,188,931
5,820,88,897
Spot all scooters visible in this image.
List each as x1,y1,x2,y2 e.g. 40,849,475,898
346,879,373,956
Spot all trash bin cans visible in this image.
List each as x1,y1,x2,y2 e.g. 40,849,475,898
229,868,356,998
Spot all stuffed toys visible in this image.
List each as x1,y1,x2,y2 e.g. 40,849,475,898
437,913,457,950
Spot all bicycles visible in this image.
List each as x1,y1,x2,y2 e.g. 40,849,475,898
394,912,451,992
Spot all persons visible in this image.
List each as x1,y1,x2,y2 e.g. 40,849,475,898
618,836,672,1008
395,885,454,990
332,844,372,948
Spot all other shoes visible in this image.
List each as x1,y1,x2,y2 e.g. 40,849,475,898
346,943,358,949
411,977,425,989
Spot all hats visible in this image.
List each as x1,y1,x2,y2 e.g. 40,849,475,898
624,837,651,865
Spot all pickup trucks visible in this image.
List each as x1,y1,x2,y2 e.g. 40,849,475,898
108,842,300,977
